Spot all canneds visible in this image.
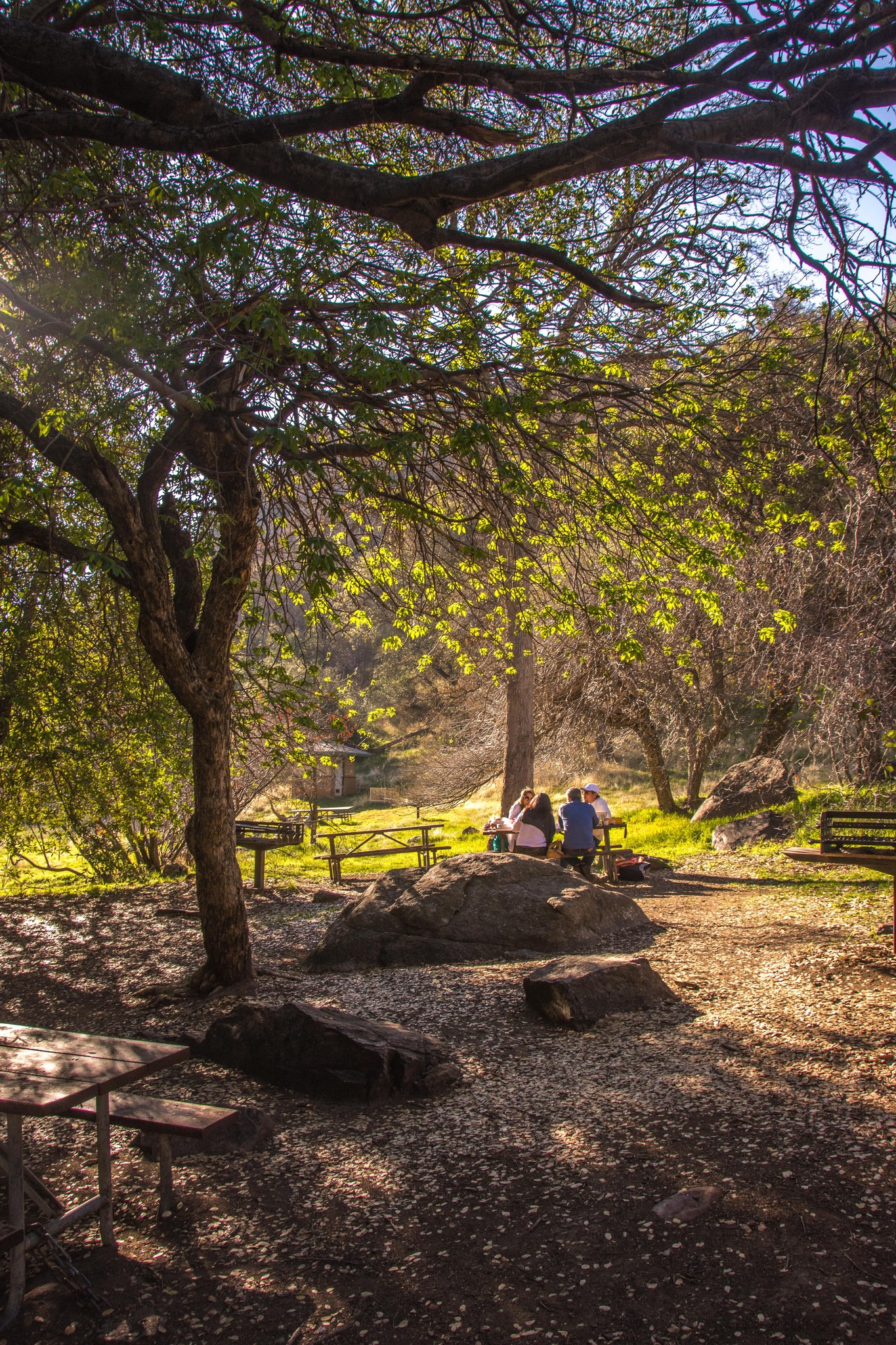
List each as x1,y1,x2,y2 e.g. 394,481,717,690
600,815,605,825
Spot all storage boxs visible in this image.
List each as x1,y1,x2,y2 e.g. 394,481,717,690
603,817,622,824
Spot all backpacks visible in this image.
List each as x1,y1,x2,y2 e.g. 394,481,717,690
618,862,650,882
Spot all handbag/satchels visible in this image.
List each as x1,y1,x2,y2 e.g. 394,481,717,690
546,840,564,859
493,834,508,852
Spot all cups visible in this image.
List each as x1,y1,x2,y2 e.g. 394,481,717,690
603,817,609,826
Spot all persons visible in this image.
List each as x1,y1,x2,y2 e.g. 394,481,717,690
558,787,599,884
511,793,556,857
580,783,612,848
506,788,536,852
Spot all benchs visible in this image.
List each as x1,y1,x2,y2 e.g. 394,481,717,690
287,812,356,818
314,844,451,859
62,1091,239,1225
486,844,634,859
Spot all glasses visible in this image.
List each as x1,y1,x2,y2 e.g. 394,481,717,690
582,790,595,794
524,796,533,801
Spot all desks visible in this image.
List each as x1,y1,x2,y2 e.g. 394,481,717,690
288,806,354,821
0,1023,190,1330
483,823,627,882
313,824,444,884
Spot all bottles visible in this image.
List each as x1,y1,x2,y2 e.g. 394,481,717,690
600,814,605,822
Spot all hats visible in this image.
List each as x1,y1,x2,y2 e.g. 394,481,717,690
580,783,600,794
